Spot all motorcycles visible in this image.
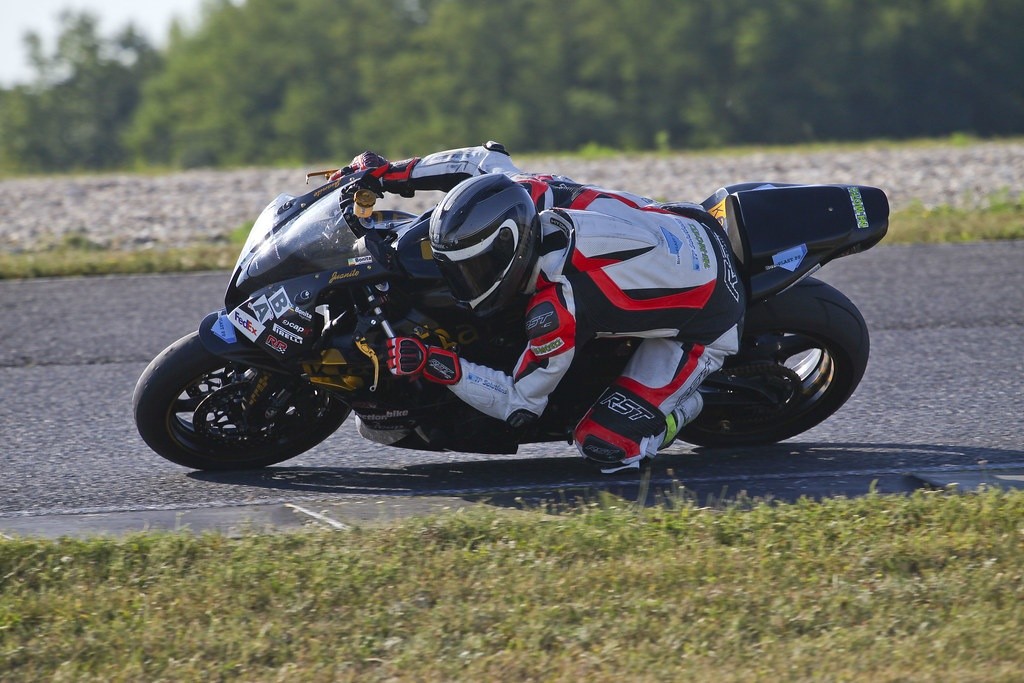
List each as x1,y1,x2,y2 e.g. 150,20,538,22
127,160,890,457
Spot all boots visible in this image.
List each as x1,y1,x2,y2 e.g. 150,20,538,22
659,392,706,455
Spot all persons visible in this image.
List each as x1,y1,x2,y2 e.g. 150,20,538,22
326,139,747,473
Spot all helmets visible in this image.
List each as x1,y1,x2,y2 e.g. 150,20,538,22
429,174,540,319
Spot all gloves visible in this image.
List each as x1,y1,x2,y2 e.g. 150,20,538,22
383,339,462,386
349,148,419,198
